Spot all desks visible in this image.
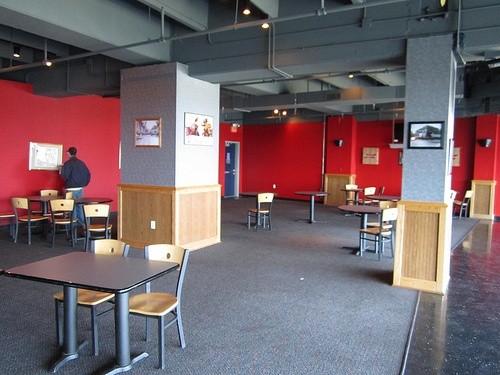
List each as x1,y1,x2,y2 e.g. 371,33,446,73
340,188,363,205
367,195,400,205
338,204,383,256
29,196,62,238
75,197,112,242
295,191,331,225
1,251,180,375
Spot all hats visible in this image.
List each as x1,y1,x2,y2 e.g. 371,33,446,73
66,147,77,153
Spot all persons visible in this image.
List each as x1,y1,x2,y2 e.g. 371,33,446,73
61,147,90,230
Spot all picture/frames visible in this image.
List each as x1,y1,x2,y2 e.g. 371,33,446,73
134,118,162,148
408,121,444,149
184,113,215,147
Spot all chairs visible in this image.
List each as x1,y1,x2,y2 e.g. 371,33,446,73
246,192,274,231
130,243,189,370
52,238,133,358
451,190,475,219
0,189,114,255
345,185,397,259
